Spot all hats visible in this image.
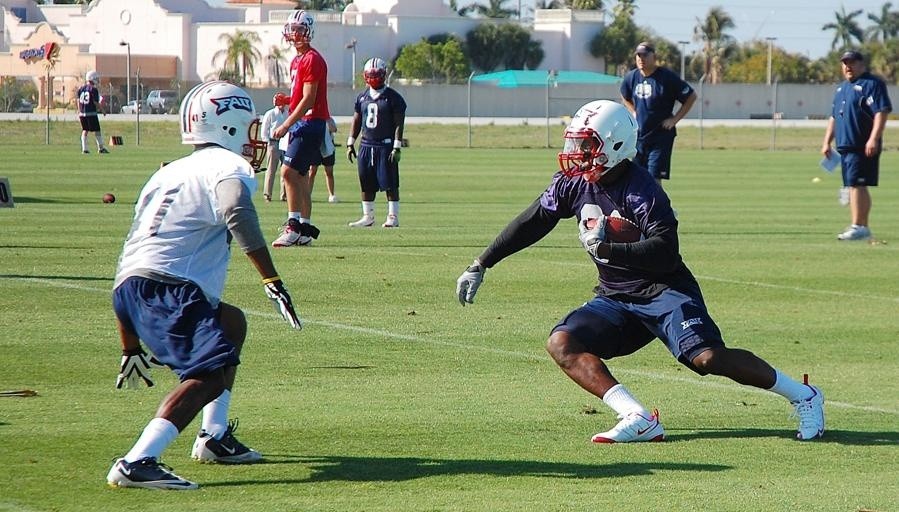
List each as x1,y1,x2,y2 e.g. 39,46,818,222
840,50,863,61
634,44,654,55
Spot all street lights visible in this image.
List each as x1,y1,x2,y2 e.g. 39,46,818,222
678,39,690,84
345,39,360,88
116,40,133,108
764,36,779,84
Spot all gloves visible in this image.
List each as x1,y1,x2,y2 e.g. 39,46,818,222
345,144,358,163
388,148,401,164
578,215,610,268
262,275,303,331
457,260,484,306
116,346,164,390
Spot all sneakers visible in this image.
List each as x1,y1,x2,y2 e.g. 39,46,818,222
790,382,825,441
591,413,664,442
272,218,301,247
349,216,375,227
107,457,199,490
83,150,90,154
382,215,399,227
838,224,870,240
191,424,261,464
99,148,109,153
297,222,320,246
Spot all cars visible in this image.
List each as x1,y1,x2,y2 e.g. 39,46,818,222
121,99,145,114
14,97,34,112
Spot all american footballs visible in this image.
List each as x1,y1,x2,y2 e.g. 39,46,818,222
585,217,641,244
103,193,115,203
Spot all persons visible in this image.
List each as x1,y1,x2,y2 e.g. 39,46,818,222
107,81,302,490
822,51,892,240
272,10,328,246
458,99,826,442
75,71,110,154
346,57,408,226
261,92,289,202
308,116,336,202
620,41,698,189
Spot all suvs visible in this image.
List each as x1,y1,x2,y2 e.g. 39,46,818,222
146,90,178,114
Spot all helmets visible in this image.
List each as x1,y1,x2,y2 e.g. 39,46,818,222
363,58,387,90
283,10,315,45
177,79,268,174
86,71,100,85
559,100,638,184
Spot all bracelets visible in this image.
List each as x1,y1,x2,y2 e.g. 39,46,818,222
346,137,355,146
280,124,288,132
393,140,401,149
